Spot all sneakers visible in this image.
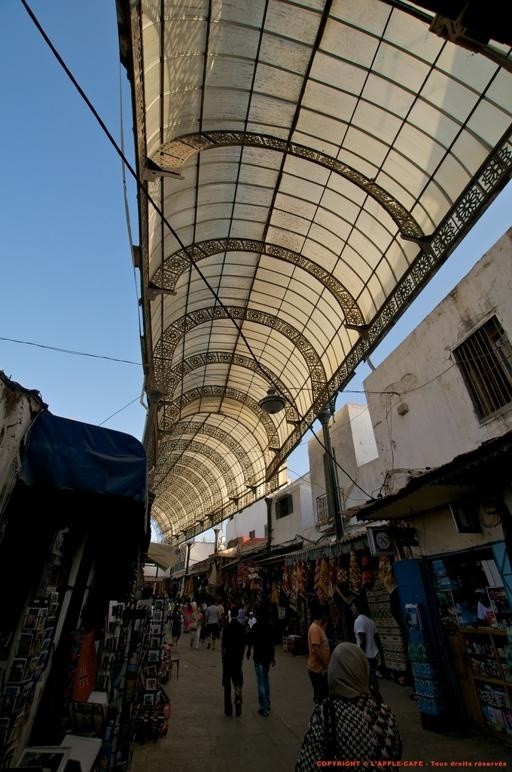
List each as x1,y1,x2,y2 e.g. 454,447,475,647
190,639,216,650
224,700,272,717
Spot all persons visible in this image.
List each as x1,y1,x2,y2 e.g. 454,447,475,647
172,593,389,718
294,640,403,772
474,587,495,626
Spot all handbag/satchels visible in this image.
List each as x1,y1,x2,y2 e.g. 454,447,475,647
323,695,346,772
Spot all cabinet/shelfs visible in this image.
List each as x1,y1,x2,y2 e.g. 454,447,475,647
449,629,512,743
